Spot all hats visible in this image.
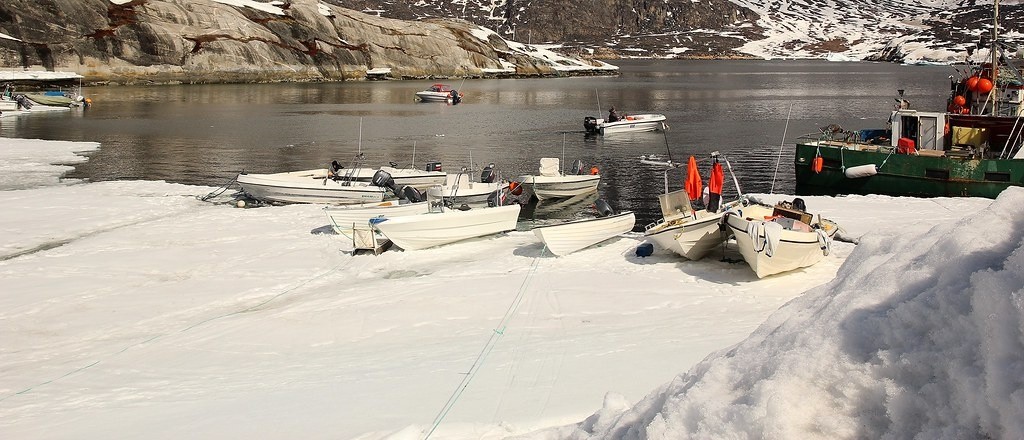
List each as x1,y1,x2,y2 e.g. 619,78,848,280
609,108,615,112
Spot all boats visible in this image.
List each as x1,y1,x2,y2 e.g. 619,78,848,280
373,203,521,250
793,0,1024,200
413,83,463,105
583,113,669,136
532,199,636,257
321,202,430,234
515,158,601,201
334,116,447,196
725,197,840,278
424,170,510,203
234,169,387,205
0,99,19,111
643,149,741,262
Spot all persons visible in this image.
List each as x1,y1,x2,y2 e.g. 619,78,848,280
609,107,622,122
